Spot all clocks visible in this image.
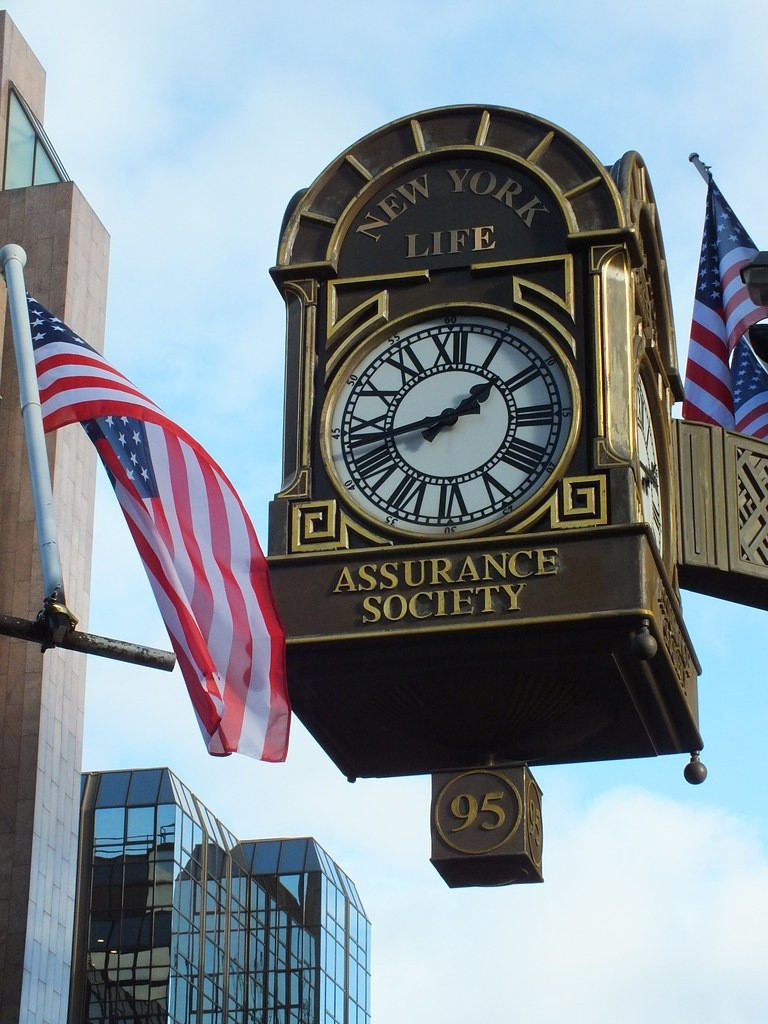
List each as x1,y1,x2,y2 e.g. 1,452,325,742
248,103,708,889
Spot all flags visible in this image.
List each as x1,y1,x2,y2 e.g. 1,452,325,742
678,173,768,438
10,274,299,767
